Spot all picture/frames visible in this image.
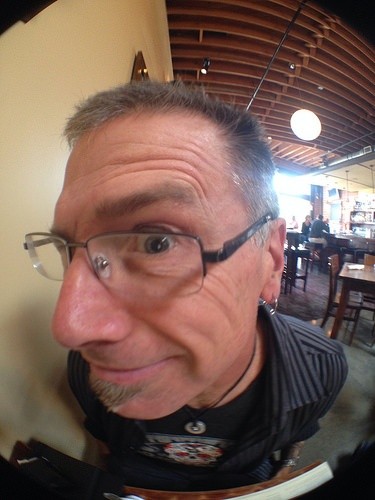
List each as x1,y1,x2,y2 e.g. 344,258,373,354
131,51,151,82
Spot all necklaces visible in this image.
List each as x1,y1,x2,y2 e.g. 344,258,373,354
181,331,258,435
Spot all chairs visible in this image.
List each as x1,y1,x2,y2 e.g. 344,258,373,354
320,254,363,347
281,232,309,294
347,254,375,319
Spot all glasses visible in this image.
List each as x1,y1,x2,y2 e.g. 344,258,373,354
23,212,274,299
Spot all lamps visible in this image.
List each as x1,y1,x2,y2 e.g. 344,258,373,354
290,68,321,141
201,58,210,75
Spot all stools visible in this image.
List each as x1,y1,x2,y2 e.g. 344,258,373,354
304,243,323,272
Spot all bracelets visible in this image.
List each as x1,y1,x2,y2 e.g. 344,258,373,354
283,454,306,469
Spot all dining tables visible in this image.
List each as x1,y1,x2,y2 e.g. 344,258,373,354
330,262,375,340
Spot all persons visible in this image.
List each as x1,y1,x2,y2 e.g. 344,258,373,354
23,80,348,491
288,212,299,236
301,209,330,252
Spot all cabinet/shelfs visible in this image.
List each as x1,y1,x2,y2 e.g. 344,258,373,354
350,210,375,237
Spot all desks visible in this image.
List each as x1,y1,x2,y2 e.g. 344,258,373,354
284,244,309,291
